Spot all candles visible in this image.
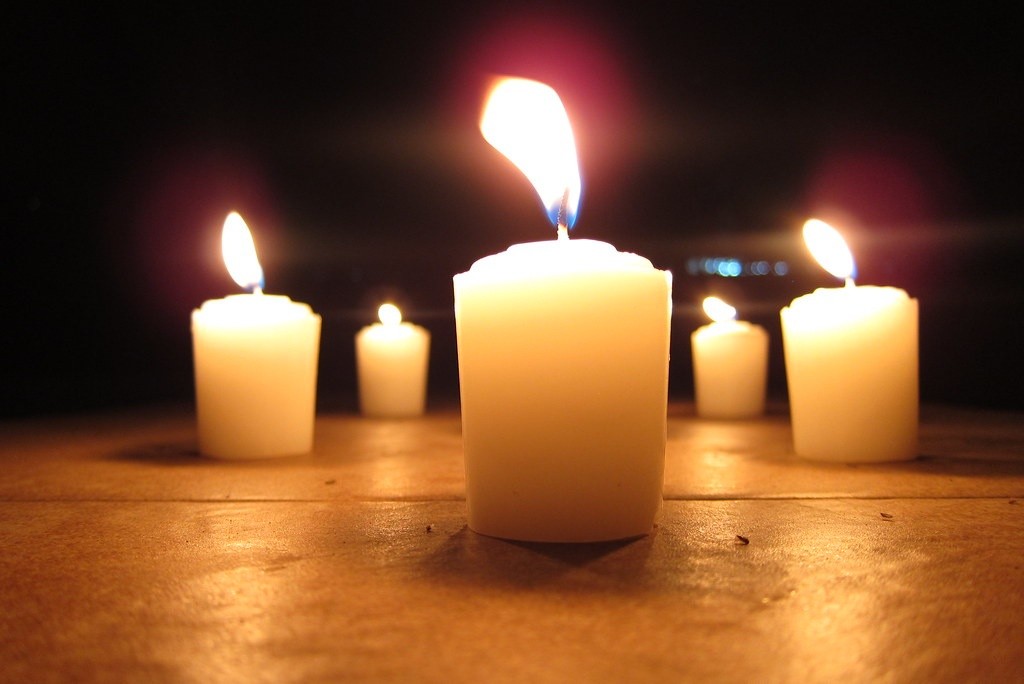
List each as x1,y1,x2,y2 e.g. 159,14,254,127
354,323,429,419
690,320,769,419
191,294,321,460
780,286,920,462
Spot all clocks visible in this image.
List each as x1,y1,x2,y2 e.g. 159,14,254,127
453,240,673,543
